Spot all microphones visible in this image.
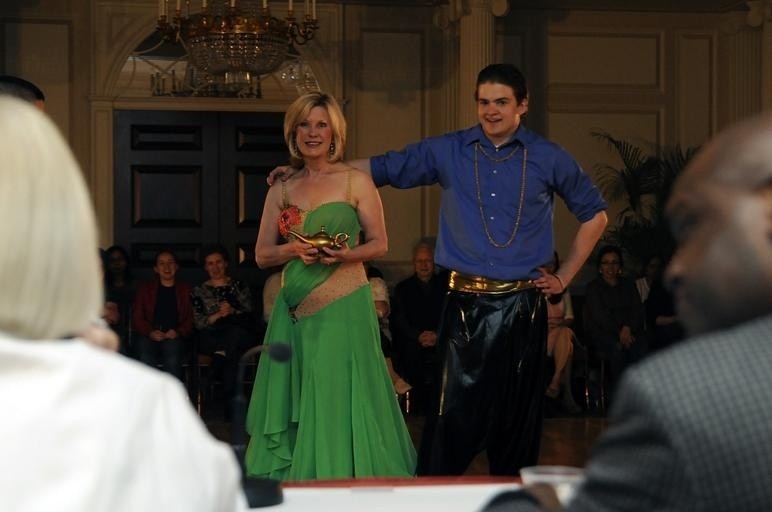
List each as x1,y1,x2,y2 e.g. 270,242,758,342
234,342,292,508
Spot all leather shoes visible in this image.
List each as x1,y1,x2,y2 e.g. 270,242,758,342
546,385,561,399
394,380,419,399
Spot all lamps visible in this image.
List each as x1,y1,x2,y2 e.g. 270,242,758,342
155,0,320,90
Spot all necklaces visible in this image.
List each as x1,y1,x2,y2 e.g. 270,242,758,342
475,142,527,248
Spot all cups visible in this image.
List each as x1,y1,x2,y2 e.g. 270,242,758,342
519,464,589,503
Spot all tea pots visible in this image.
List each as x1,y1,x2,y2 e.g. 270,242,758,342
285,225,350,251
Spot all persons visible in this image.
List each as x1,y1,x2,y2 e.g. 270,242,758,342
0,94,248,512
242,91,418,483
264,62,609,475
100,246,248,420
362,242,449,412
519,113,772,512
538,246,683,413
